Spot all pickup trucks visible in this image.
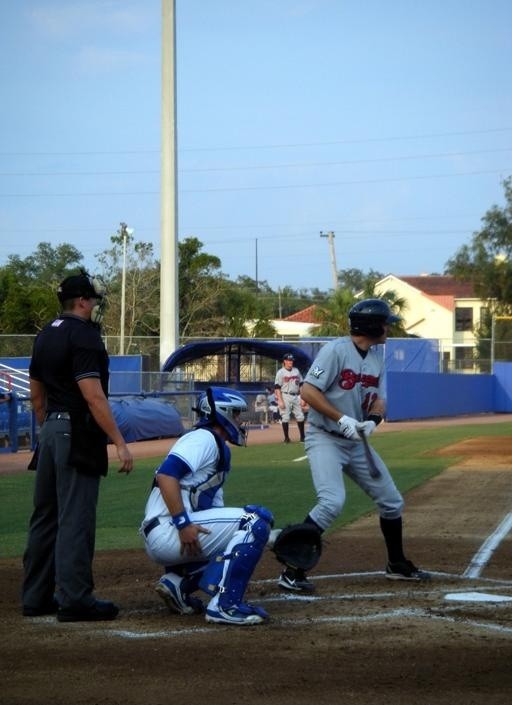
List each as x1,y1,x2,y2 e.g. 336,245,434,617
0,392,40,449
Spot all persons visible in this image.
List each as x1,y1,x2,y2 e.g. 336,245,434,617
277,299,430,593
274,353,304,443
138,386,275,626
22,275,134,621
255,387,309,424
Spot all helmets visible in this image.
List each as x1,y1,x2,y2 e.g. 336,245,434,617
192,386,250,448
349,299,402,337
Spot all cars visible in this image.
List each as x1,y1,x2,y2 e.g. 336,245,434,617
157,380,200,415
250,391,278,424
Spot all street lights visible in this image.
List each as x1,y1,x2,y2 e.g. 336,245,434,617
486,296,499,375
120,225,134,355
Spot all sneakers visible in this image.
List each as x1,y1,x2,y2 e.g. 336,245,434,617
23,592,57,616
56,600,120,622
277,570,315,592
385,561,432,581
205,604,264,625
155,575,195,615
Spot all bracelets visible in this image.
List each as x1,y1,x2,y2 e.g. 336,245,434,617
174,512,191,529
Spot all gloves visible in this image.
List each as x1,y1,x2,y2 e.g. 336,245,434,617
337,415,376,441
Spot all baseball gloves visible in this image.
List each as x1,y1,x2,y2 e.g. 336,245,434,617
273,523,321,569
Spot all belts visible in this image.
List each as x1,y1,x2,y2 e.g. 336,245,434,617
284,392,299,395
48,412,70,420
144,520,160,537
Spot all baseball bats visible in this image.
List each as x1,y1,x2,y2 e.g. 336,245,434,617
359,430,380,478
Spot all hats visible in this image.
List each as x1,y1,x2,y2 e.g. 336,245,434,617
57,277,103,304
283,353,295,361
